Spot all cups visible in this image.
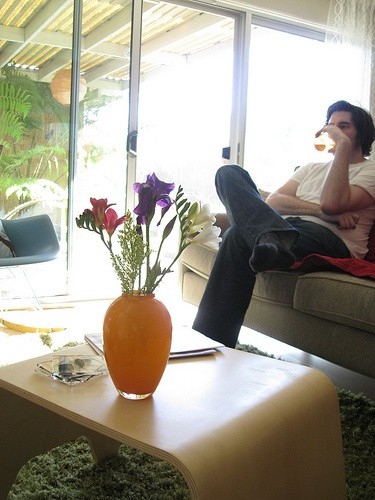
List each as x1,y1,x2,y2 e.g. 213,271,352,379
314,131,335,152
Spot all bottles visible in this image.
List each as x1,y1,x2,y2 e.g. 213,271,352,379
103,290,173,400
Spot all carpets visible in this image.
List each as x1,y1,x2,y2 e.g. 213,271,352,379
0,341,375,500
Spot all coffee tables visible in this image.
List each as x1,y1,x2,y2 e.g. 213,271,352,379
0,343,347,500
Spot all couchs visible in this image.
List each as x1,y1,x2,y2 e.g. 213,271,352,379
179,213,375,379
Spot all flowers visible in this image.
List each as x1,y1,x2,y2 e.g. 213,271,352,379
76,172,222,296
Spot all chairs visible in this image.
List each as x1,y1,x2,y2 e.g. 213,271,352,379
0,214,68,333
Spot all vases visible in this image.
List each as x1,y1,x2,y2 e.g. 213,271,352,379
103,290,172,400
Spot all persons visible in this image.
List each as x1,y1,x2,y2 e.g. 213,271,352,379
192,100,375,349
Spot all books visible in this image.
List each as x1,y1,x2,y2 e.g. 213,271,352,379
85,324,225,359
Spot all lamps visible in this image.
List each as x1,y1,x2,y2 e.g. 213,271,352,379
50,69,87,106
